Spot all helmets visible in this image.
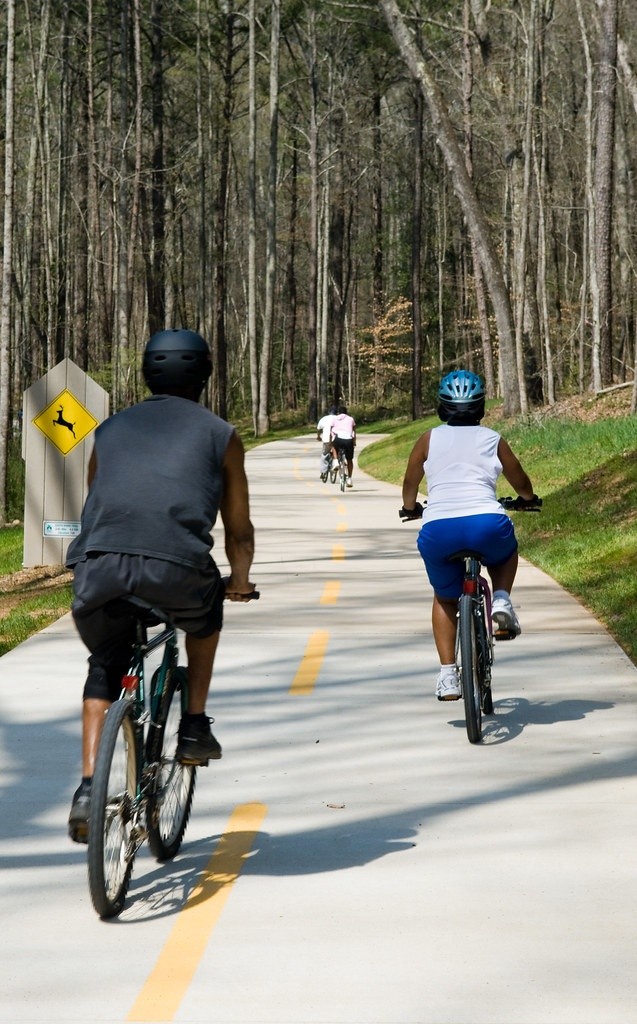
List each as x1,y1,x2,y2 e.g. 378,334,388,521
142,329,213,393
435,371,486,421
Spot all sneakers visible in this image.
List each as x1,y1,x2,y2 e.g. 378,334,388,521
434,669,460,696
69,784,92,844
490,600,521,637
177,716,222,765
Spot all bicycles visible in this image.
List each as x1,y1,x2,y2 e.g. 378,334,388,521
73,590,262,920
318,437,354,493
399,495,544,743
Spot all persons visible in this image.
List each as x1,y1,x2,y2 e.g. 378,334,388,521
400,368,539,700
67,329,258,842
316,406,357,487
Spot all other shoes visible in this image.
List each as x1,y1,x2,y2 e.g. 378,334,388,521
331,465,339,472
346,479,353,487
320,473,325,479
